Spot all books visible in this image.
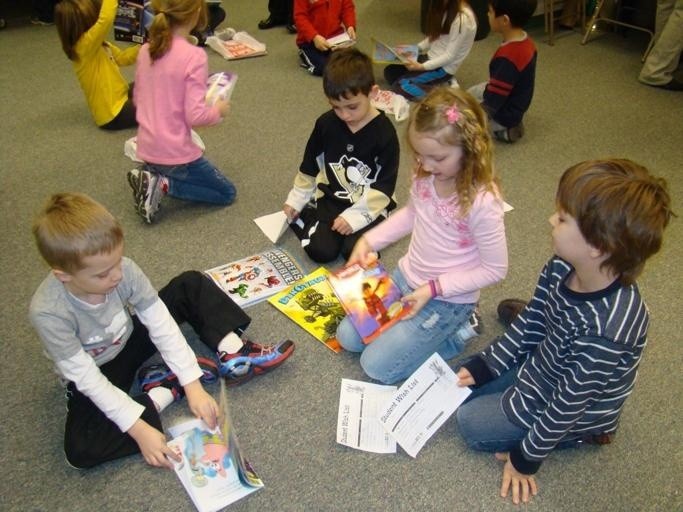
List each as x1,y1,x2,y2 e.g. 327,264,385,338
161,376,267,512
113,0,155,46
202,245,412,357
367,35,418,68
325,31,356,52
202,70,238,114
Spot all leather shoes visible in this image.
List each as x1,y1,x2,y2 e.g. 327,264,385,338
659,79,682,91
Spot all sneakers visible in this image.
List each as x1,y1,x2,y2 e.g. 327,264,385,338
495,121,524,143
216,339,294,386
138,357,218,401
454,300,482,341
498,299,525,325
127,169,164,224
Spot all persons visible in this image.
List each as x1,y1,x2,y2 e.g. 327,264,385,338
633,0,682,92
52,0,142,133
124,1,238,227
453,156,679,507
291,1,359,78
380,1,481,106
333,80,512,388
280,44,401,265
256,1,300,37
463,0,539,148
27,192,292,468
